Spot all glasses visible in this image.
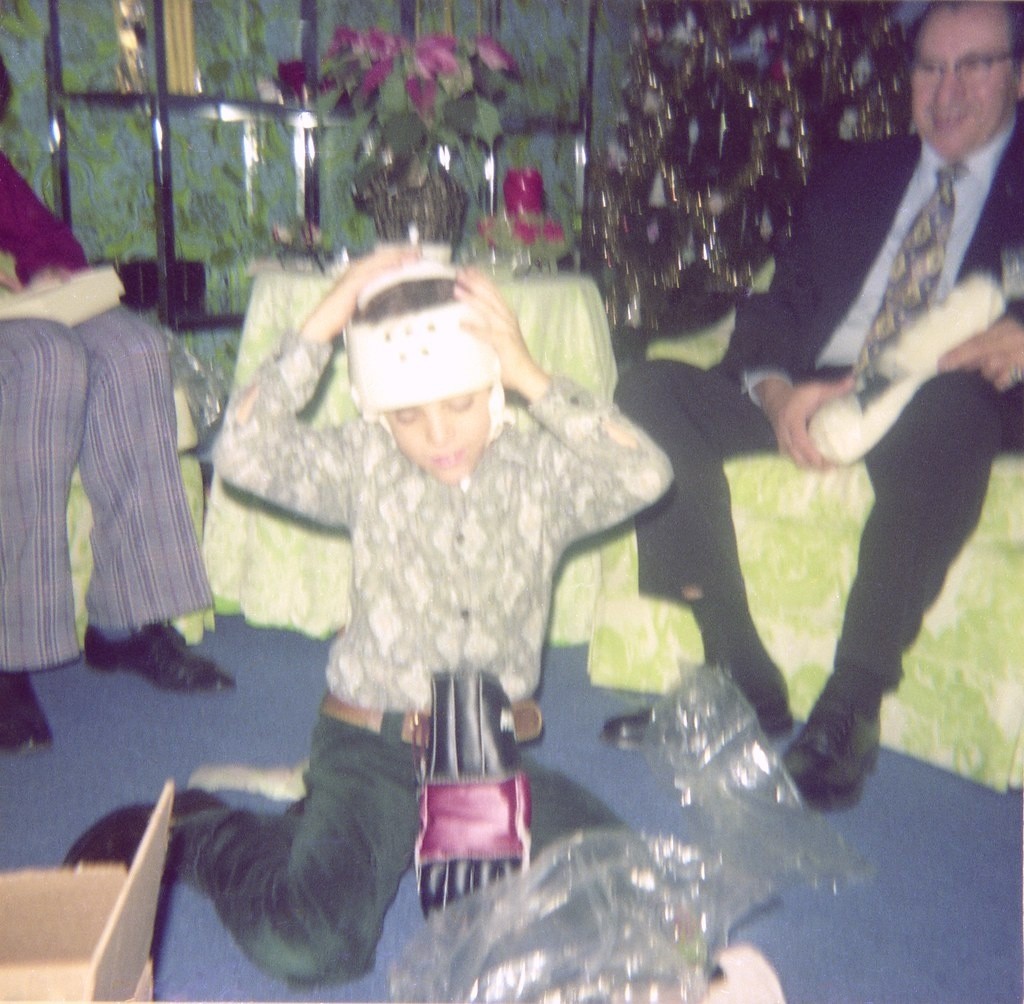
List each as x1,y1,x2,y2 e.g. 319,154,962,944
909,50,1019,84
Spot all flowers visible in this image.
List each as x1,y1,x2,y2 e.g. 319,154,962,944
479,166,573,267
273,24,521,160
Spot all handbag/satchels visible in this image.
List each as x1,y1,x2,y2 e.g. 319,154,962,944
1,265,127,328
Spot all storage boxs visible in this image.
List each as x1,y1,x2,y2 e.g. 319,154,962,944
0,777,178,1004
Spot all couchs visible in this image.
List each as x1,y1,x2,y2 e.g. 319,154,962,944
1,250,217,656
590,255,1024,791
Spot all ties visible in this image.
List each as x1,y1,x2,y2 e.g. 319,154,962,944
845,164,973,409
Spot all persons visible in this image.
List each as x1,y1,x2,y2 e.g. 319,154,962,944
615,0,1022,811
0,55,237,759
61,242,676,987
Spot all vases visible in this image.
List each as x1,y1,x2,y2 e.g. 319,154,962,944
372,146,463,266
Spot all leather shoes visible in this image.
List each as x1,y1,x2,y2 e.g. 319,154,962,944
0,669,52,755
781,673,880,809
602,651,794,752
86,619,235,689
61,789,228,869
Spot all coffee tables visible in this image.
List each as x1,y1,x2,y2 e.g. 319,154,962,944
201,259,620,644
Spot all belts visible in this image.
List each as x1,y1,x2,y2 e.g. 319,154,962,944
320,691,542,748
